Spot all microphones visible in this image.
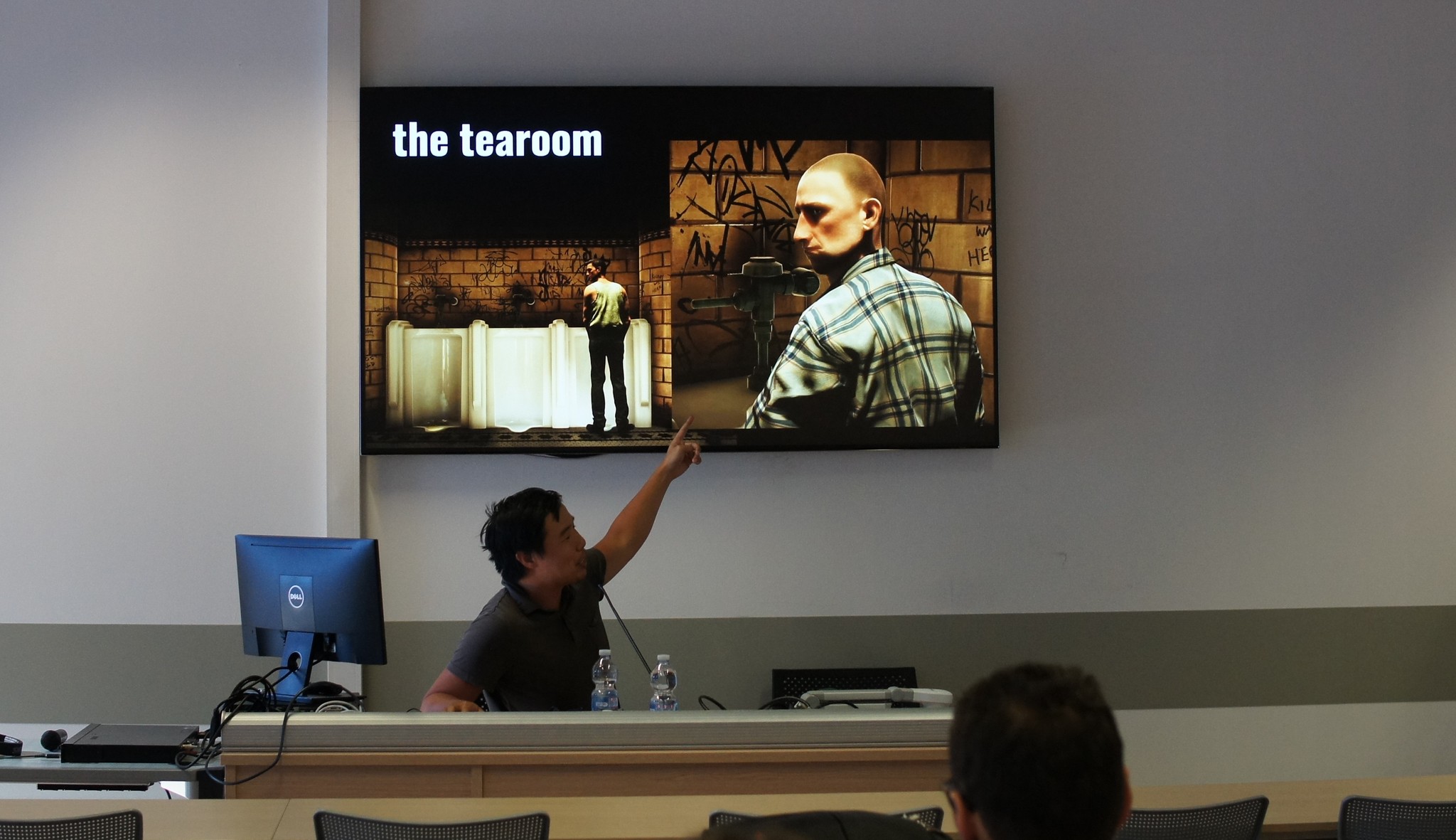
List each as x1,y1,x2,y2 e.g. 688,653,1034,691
40,727,67,750
589,571,651,676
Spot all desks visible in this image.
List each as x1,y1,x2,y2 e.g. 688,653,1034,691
221,711,955,800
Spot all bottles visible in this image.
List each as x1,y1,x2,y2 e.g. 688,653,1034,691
591,649,618,711
649,654,678,711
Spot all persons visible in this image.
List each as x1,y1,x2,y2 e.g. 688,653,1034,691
945,660,1133,840
418,414,702,713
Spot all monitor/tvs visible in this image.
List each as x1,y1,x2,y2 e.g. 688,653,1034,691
355,83,1003,456
234,534,387,701
